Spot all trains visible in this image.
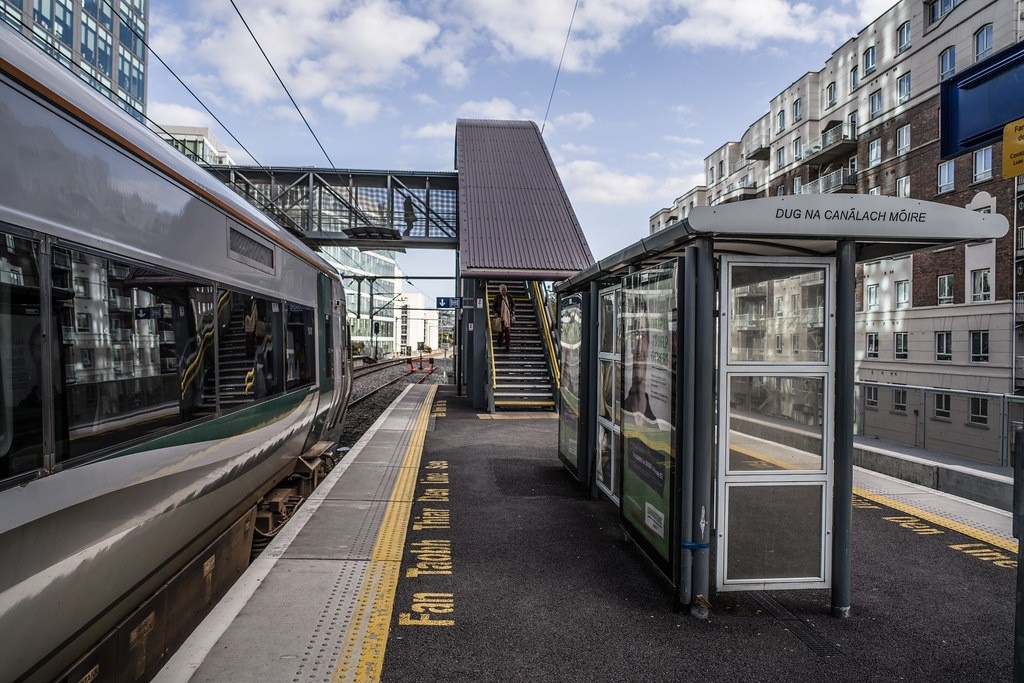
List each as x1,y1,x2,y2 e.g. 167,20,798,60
0,21,357,683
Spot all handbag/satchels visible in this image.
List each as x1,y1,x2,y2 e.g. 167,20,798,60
511,313,516,324
492,317,502,332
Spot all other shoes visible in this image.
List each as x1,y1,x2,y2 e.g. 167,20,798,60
506,349,510,352
498,343,502,347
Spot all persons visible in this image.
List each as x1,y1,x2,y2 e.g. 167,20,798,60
403,196,414,236
493,284,515,352
621,294,657,421
243,295,259,355
